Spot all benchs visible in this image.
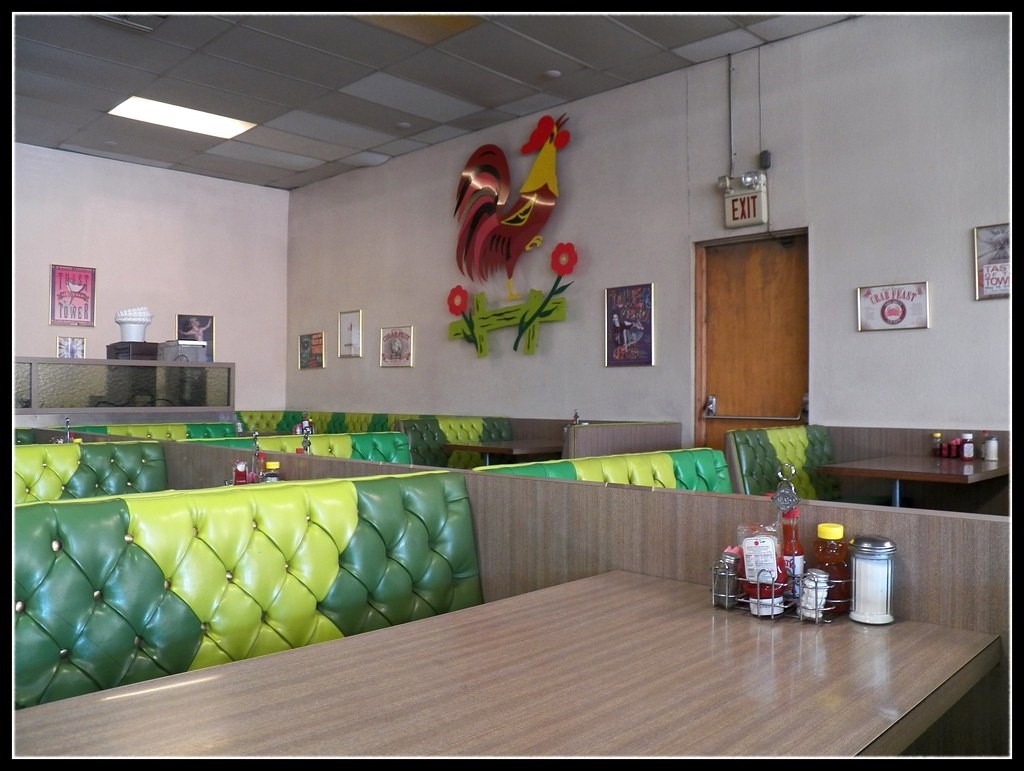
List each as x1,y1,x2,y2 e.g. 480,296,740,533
16,408,916,714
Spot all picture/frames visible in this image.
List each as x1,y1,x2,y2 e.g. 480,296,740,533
296,331,324,369
337,308,361,358
56,336,86,360
379,325,413,367
604,282,655,369
973,223,1010,301
176,314,215,362
855,282,929,332
50,264,95,327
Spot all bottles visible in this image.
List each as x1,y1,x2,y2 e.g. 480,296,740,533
737,522,787,616
712,552,742,611
777,507,805,600
932,433,999,461
230,453,282,488
292,410,315,436
813,522,854,614
797,568,831,620
845,535,896,626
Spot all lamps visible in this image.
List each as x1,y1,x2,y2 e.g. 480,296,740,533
718,171,759,192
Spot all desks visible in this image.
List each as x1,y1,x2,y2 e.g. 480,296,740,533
15,570,1004,759
818,457,1010,507
439,441,565,466
